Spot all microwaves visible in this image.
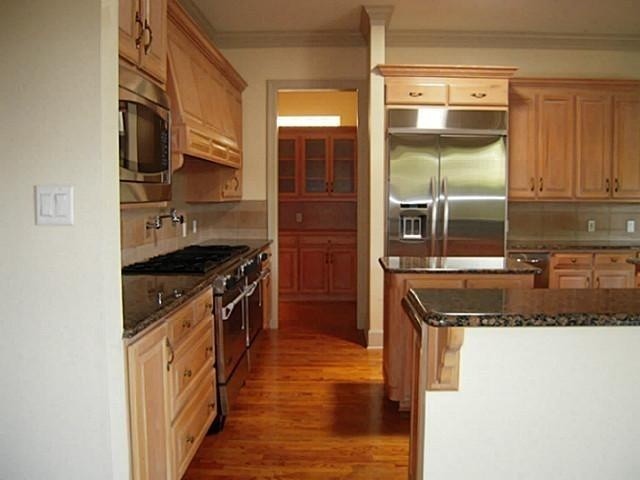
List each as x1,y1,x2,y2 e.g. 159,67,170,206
119,63,173,205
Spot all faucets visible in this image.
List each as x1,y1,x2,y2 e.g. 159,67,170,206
146,208,179,229
626,256,640,265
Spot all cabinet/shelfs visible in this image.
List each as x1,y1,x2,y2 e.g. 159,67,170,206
278,125,298,204
402,275,525,412
298,123,357,204
187,77,245,205
385,76,509,108
296,226,357,303
274,229,299,303
119,0,172,91
507,83,576,200
167,283,224,480
546,251,637,291
125,319,179,480
572,84,640,200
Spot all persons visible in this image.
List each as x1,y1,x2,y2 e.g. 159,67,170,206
224,175,241,198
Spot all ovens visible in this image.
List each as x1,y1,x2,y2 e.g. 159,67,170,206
217,263,271,420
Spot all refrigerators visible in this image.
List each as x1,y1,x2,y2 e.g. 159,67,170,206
385,108,506,258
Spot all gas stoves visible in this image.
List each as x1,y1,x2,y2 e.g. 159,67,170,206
121,242,268,291
